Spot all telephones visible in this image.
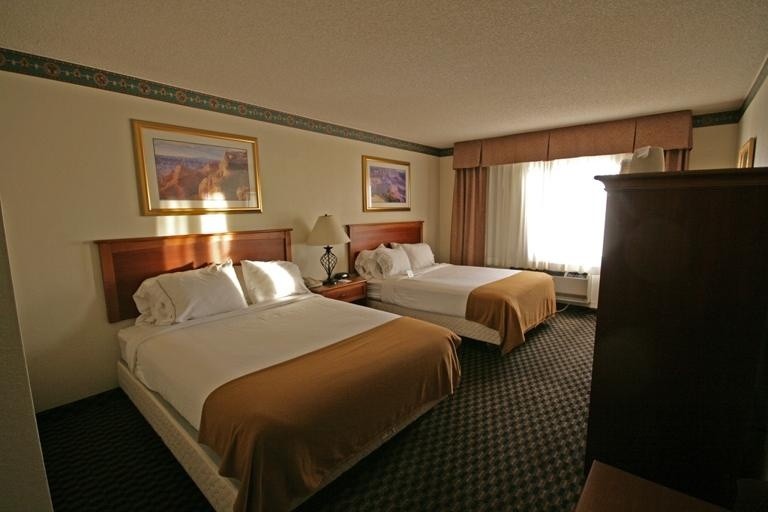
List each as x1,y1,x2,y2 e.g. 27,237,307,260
302,276,323,288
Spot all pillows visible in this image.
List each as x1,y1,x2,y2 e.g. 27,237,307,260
389,241,435,274
144,258,250,328
373,243,416,278
355,243,387,279
239,257,312,306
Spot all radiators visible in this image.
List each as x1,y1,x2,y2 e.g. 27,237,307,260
511,266,593,307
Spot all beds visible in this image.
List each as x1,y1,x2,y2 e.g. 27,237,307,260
83,226,466,511
344,219,560,359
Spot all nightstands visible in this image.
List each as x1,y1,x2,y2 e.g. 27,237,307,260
303,273,368,304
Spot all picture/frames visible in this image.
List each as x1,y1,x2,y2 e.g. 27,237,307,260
735,136,756,170
360,153,413,214
126,116,265,216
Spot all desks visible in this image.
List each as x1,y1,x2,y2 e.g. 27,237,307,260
578,460,722,511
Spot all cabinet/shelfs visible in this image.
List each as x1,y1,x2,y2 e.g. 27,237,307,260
579,170,768,511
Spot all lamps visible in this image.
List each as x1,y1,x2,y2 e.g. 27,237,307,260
302,212,351,286
